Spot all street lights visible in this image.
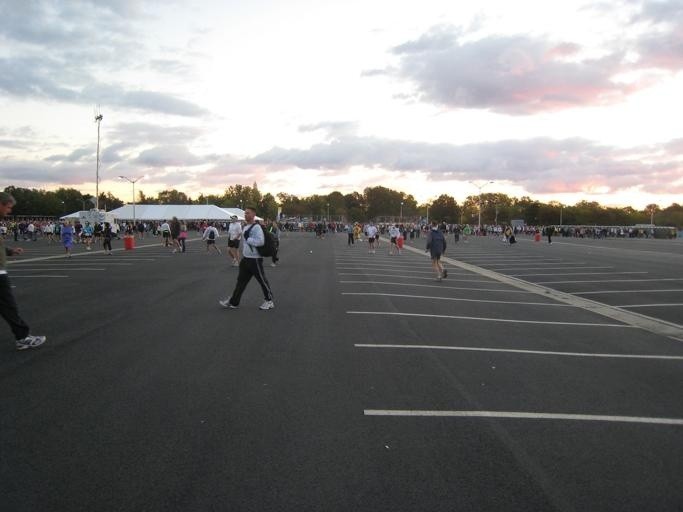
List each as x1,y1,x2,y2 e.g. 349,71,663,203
118,173,143,227
558,206,566,225
325,203,331,222
90,113,102,212
359,203,362,214
399,202,404,224
649,207,653,225
468,180,494,228
239,198,242,209
205,196,208,204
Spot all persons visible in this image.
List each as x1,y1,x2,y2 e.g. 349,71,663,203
256,219,449,282
1,215,248,266
449,224,675,248
219,208,275,310
0,193,46,351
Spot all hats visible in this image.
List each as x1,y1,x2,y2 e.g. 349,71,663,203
229,214,238,220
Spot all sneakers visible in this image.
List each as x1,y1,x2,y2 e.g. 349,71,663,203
367,248,402,257
270,258,280,267
230,257,240,267
432,268,448,281
63,247,114,258
159,242,186,253
258,299,275,310
218,295,241,309
14,332,46,351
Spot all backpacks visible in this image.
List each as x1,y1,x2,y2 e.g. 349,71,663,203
208,226,216,239
246,221,278,258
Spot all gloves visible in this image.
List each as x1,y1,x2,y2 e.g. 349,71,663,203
243,230,249,240
